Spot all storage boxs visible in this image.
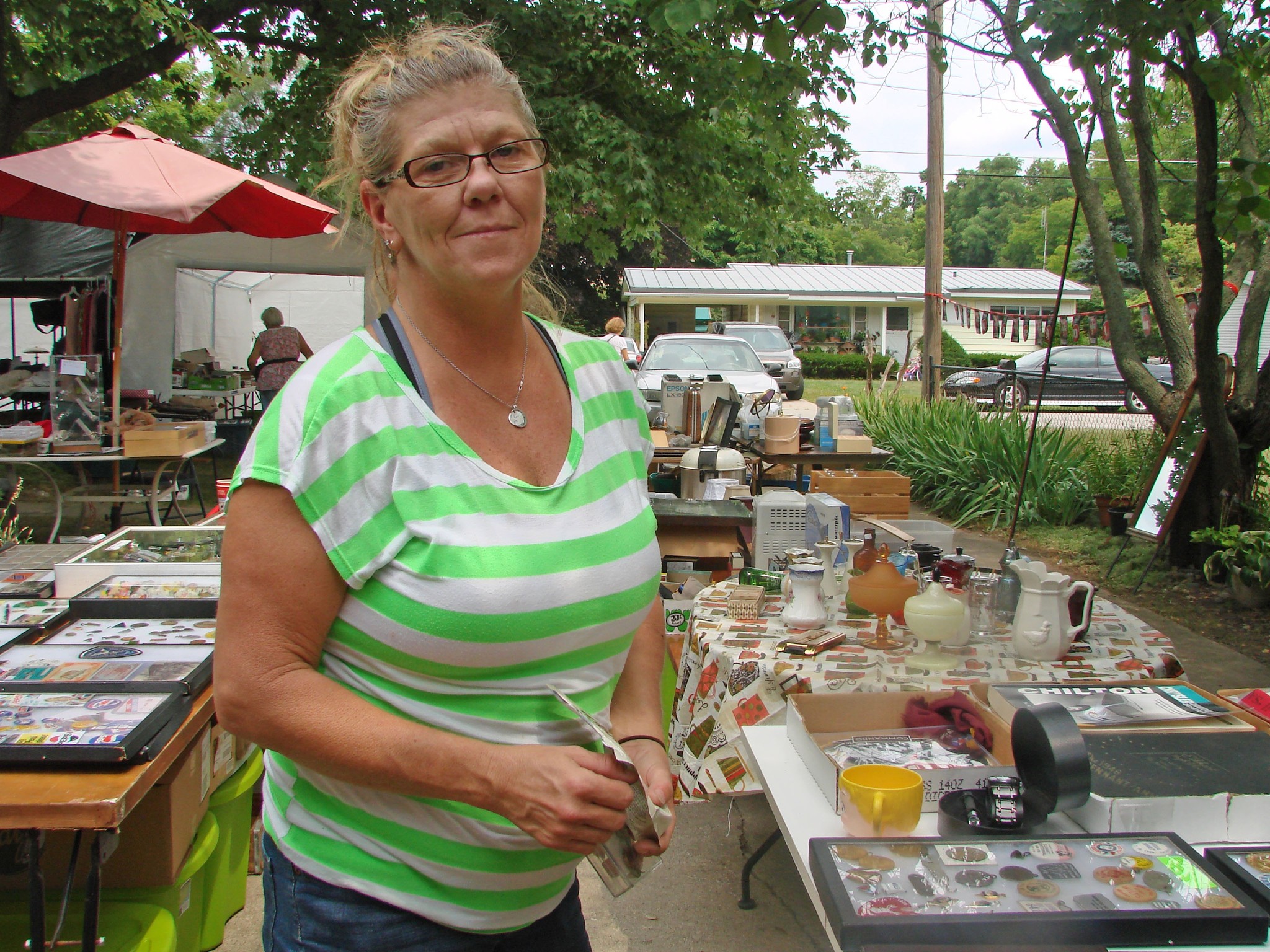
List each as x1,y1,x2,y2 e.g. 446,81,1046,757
811,470,911,521
124,420,206,457
851,519,954,562
805,491,850,568
172,349,251,390
785,686,1270,834
813,417,872,454
0,709,265,952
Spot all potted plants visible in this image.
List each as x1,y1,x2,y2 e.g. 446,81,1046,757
1089,428,1167,534
1189,522,1270,609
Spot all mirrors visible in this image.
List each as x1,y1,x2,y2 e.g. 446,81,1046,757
1124,353,1233,542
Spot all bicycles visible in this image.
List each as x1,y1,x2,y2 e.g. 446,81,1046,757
896,358,923,382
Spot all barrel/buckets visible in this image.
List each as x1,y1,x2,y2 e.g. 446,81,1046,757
216,479,232,510
761,415,801,454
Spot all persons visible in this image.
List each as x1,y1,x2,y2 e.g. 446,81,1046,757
599,317,632,372
247,307,314,413
213,19,677,952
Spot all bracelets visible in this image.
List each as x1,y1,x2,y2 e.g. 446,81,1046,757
617,735,666,752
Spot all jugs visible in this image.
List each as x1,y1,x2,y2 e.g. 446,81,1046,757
1010,559,1098,661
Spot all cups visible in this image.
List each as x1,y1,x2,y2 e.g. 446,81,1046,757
969,575,999,632
838,764,923,838
702,479,755,543
888,542,953,594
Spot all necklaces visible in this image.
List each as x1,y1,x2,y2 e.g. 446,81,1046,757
396,294,528,428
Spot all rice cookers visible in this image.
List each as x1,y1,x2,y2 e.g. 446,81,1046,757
680,445,747,498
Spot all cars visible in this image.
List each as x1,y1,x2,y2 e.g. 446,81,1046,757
940,346,1175,414
621,321,805,438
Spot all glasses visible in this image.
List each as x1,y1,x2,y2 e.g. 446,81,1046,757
373,136,550,193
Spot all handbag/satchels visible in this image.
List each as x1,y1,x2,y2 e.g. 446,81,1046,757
31,300,64,326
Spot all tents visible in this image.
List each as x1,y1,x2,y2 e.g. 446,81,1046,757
120,173,392,420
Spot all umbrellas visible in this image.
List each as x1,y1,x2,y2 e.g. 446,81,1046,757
0,116,341,531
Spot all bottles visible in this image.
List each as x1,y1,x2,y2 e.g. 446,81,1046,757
903,568,966,670
738,527,917,650
938,547,976,589
996,539,1022,623
824,467,859,477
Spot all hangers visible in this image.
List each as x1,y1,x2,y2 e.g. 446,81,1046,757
60,275,110,301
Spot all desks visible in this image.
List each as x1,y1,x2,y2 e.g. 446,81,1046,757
173,385,258,419
2,438,227,542
668,569,1187,910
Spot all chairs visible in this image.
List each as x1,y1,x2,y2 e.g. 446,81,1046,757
658,354,687,369
714,355,737,371
